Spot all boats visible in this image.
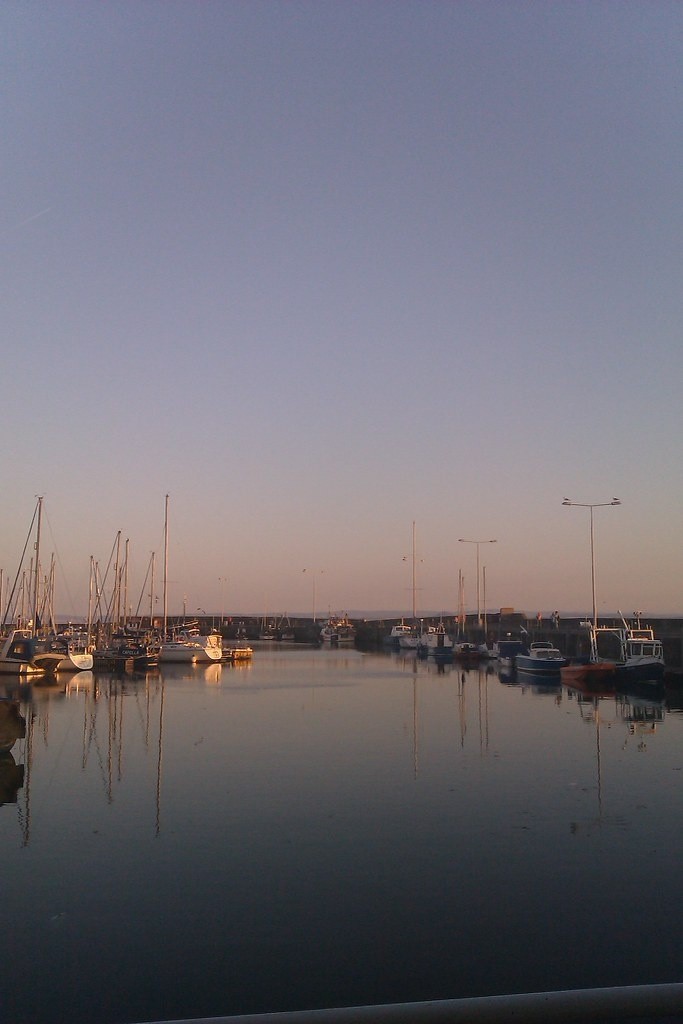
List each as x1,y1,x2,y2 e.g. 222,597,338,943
201,566,666,748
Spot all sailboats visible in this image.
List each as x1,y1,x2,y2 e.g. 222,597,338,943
0,493,252,677
0,660,252,848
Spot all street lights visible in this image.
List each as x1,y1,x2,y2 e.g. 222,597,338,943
402,555,425,617
457,538,498,628
562,496,621,629
